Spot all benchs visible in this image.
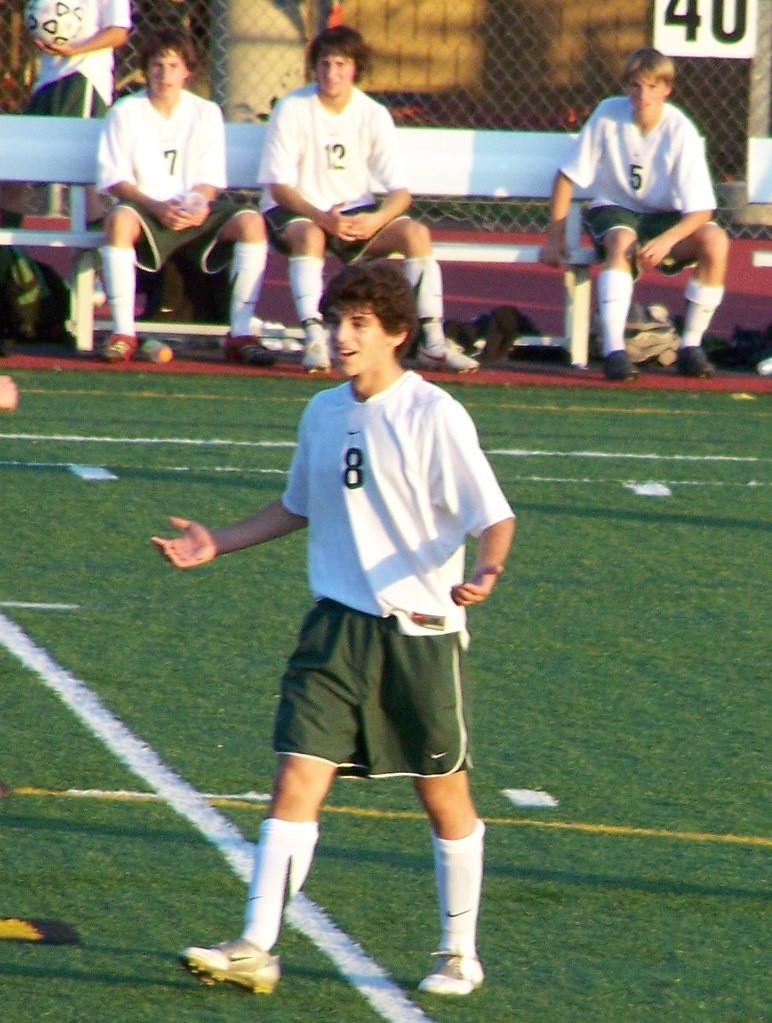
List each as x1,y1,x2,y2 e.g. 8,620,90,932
0,114,595,371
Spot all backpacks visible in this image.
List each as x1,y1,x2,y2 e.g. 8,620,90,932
0,209,67,346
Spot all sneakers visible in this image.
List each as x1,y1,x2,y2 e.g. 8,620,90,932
225,331,277,366
179,938,282,995
418,951,485,996
101,333,137,361
678,346,714,378
416,341,480,373
602,350,638,379
301,341,332,374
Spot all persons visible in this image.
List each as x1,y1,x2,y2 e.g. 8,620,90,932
28,0,131,187
259,24,481,373
99,28,278,363
539,49,729,383
150,264,517,993
